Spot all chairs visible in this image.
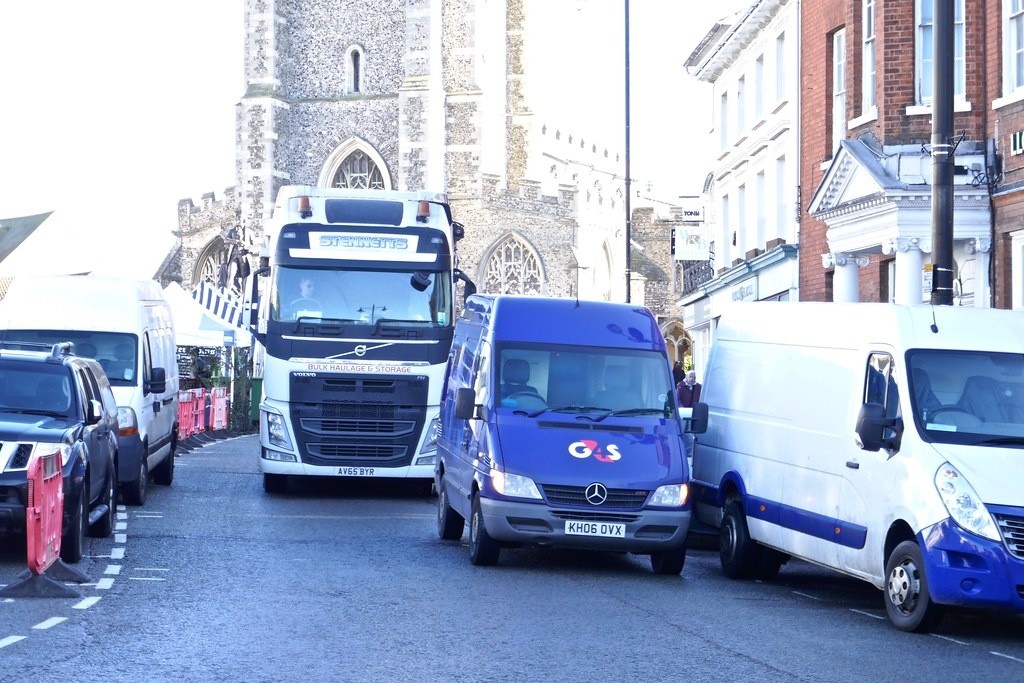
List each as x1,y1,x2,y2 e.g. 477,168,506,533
499,360,538,401
910,366,941,425
74,342,97,358
107,343,133,382
594,364,645,409
960,375,1012,424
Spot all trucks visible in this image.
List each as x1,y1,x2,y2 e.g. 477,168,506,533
242,187,481,494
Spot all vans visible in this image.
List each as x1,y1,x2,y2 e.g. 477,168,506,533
0,269,182,505
684,301,1023,633
434,293,712,576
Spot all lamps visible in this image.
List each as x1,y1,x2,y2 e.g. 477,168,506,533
881,237,895,254
821,253,834,268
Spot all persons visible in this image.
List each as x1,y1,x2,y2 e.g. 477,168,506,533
289,274,318,311
672,360,686,386
676,370,703,408
186,347,212,387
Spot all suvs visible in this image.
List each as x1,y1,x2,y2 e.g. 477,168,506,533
1,341,120,563
